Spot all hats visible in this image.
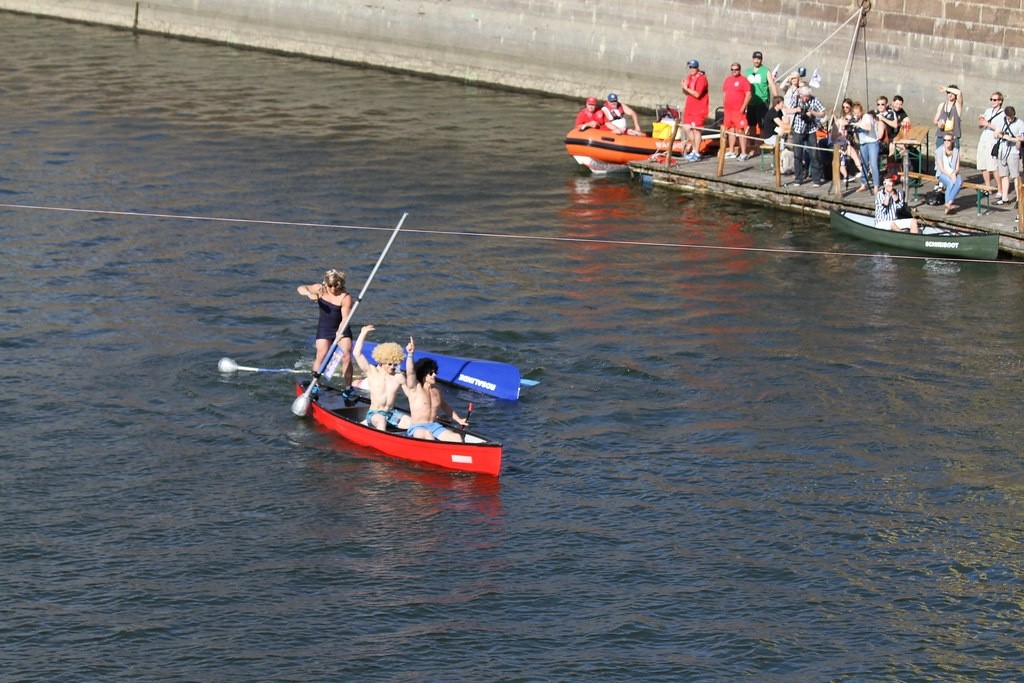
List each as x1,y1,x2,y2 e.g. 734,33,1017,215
752,51,763,59
608,94,617,101
587,97,597,105
687,59,698,67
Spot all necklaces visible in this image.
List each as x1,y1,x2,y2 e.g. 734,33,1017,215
992,106,1001,117
946,101,956,118
945,149,952,156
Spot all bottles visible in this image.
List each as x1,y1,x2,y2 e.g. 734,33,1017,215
1013,214,1020,232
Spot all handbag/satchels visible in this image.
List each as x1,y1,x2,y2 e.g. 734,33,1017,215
800,108,816,124
990,144,999,156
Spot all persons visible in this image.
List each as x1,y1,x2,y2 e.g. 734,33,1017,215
991,105,1024,209
874,178,919,234
406,335,469,443
602,94,648,137
353,324,411,432
780,72,911,193
976,92,1006,198
935,132,963,215
1014,132,1024,222
934,85,963,185
680,59,709,163
763,96,791,167
297,267,359,403
744,52,778,159
722,63,752,162
576,97,621,135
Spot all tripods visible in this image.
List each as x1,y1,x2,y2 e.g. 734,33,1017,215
828,134,874,196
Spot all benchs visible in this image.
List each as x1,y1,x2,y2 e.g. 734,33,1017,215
897,171,998,216
761,144,775,170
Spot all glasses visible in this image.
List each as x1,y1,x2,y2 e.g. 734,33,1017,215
989,98,999,101
688,66,696,69
388,363,397,366
943,139,952,142
610,101,617,102
843,107,849,108
877,103,885,106
730,68,740,71
328,284,339,288
428,370,439,375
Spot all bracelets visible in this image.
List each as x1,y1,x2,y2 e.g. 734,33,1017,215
684,86,687,91
407,353,413,357
1002,134,1003,139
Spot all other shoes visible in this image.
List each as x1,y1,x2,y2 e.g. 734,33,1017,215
856,184,878,195
794,182,800,187
943,203,954,214
984,191,1018,208
813,183,820,187
842,175,856,182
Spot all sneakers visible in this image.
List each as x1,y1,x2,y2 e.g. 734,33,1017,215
684,151,703,163
310,383,358,401
724,152,748,161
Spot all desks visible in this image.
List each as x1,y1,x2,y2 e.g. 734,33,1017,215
893,124,930,200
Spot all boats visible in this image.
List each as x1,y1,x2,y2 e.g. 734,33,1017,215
296,379,503,477
828,205,1000,260
563,125,828,172
306,334,522,402
